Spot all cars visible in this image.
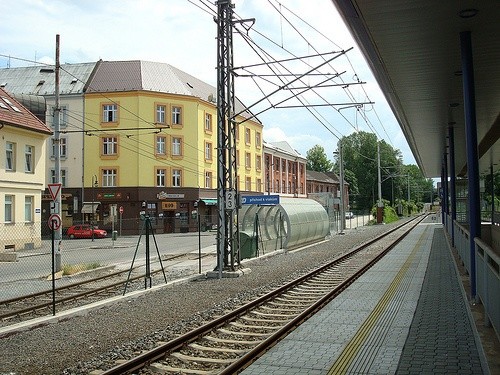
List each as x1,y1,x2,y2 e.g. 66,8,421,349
68,224,107,239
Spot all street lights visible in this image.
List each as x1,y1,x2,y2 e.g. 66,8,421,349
333,151,346,230
37,68,63,274
